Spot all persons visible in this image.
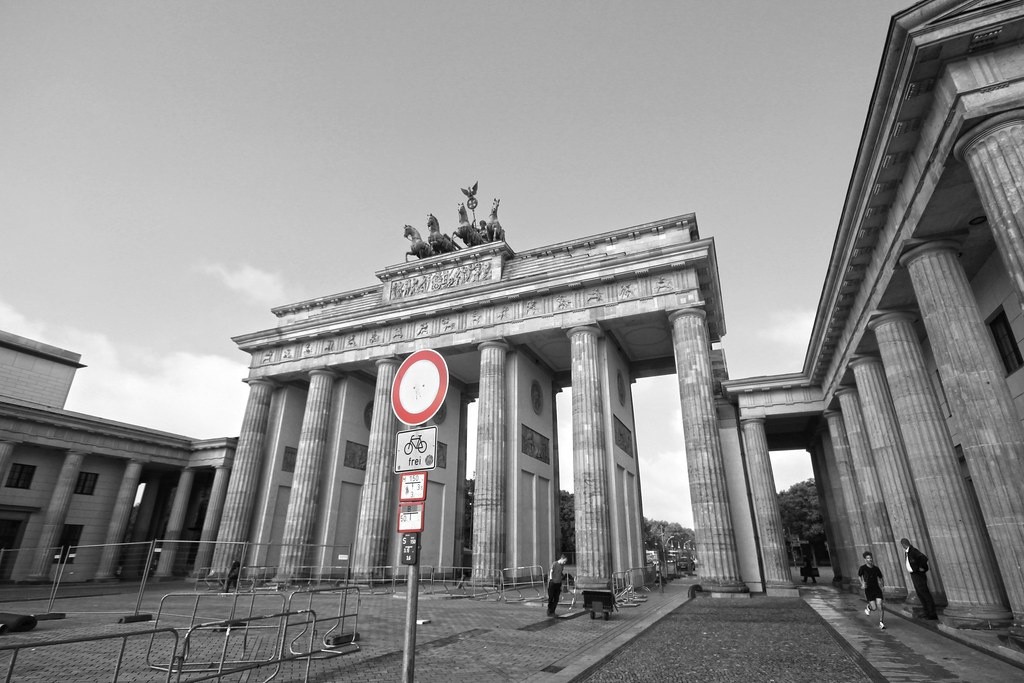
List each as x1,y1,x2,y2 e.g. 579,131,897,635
547,554,568,617
801,550,817,583
901,538,939,620
472,219,488,241
226,560,240,593
858,551,886,629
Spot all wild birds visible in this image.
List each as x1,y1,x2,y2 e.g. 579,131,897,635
460,181,478,197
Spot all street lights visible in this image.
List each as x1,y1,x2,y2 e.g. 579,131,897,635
660,524,675,579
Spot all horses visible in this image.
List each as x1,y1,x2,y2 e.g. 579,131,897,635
404,198,505,262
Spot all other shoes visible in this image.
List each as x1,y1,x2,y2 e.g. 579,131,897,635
548,612,559,618
880,621,885,629
865,605,870,616
918,613,937,620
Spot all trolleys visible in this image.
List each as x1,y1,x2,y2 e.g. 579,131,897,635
579,589,618,621
